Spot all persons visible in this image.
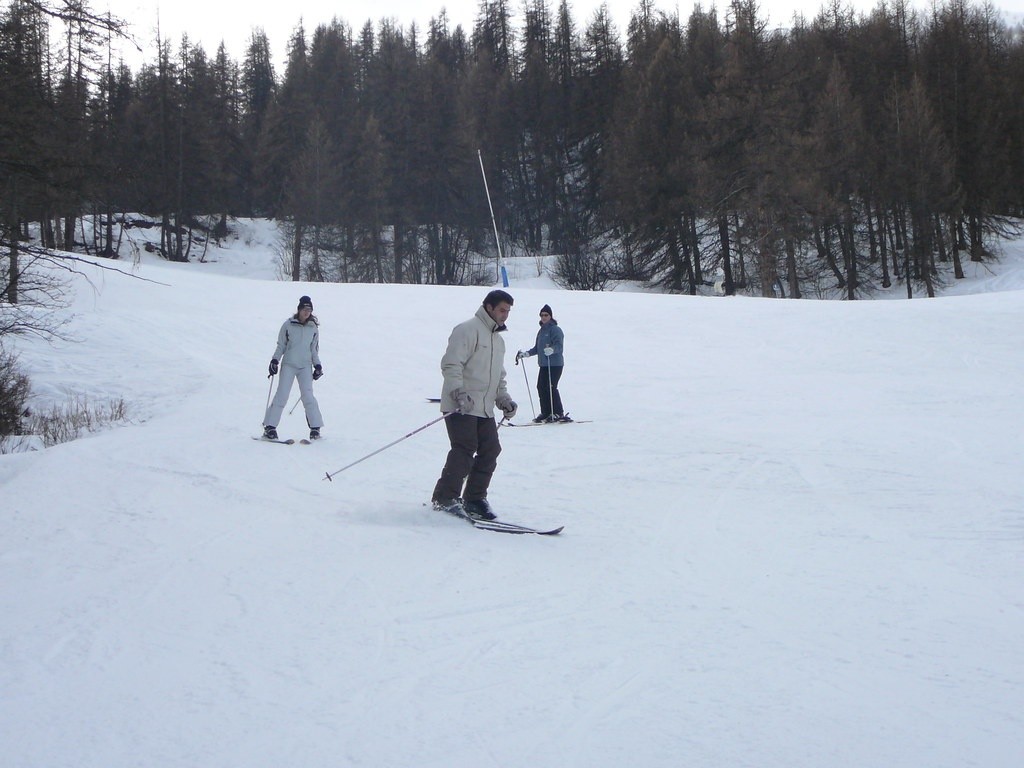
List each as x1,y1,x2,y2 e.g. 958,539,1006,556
519,304,565,423
432,289,518,520
262,295,325,440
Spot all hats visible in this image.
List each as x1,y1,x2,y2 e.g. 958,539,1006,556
540,304,552,317
299,296,313,310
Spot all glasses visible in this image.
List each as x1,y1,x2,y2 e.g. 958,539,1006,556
541,314,550,317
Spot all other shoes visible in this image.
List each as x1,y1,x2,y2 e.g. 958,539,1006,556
461,496,496,519
549,413,564,420
266,425,277,439
538,412,549,420
432,494,468,518
310,427,319,438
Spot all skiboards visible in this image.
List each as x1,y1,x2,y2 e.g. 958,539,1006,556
451,514,565,536
497,420,594,428
250,435,327,445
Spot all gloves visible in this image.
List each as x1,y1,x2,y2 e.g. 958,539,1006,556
518,352,530,359
500,400,517,421
313,365,323,380
269,360,279,374
544,347,554,356
451,387,474,416
